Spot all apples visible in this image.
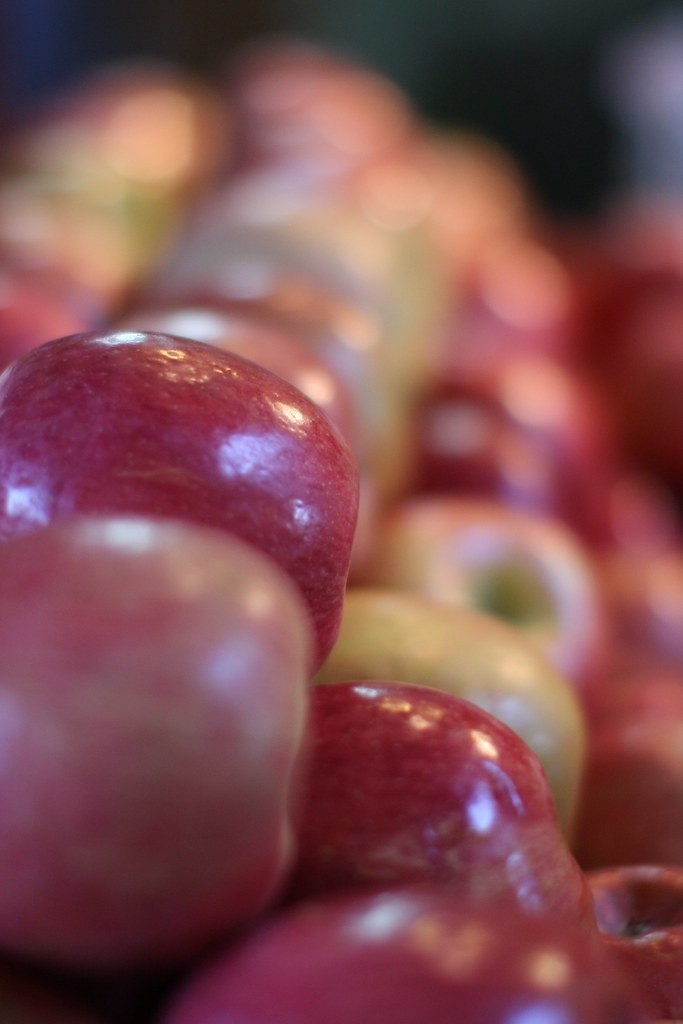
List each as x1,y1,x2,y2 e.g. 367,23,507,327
0,44,683,1024
